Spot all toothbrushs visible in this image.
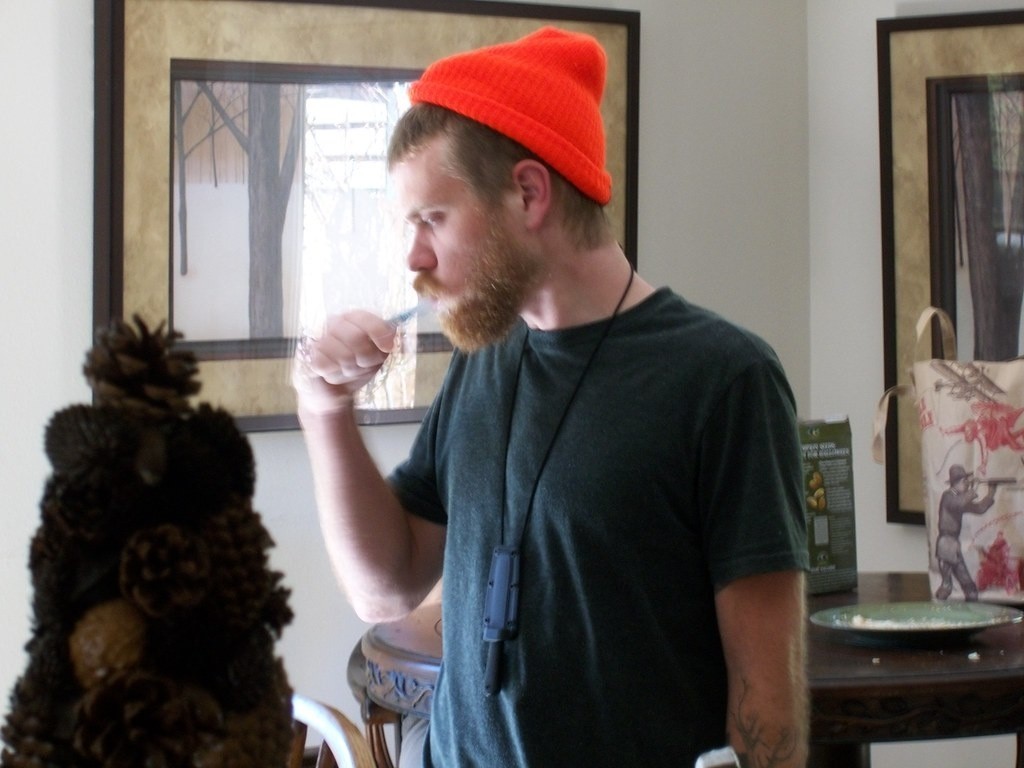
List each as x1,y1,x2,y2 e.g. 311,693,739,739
386,301,429,327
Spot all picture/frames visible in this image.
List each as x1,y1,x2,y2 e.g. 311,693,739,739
876,8,1024,527
87,1,640,434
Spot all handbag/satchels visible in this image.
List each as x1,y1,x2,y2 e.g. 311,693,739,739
874,307,1024,606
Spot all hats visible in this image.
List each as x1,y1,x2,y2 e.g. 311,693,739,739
409,25,613,205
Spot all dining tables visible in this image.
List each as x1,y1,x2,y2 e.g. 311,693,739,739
362,565,1024,768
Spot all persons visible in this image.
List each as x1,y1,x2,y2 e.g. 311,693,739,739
293,27,809,768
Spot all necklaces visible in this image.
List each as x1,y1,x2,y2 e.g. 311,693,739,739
479,266,636,697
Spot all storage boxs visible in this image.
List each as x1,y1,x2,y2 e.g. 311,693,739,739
796,415,861,595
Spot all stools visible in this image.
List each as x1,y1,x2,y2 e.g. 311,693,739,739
346,638,402,768
